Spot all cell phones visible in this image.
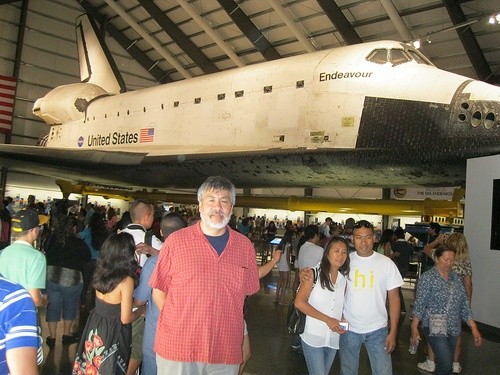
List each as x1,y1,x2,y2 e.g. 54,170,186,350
339,322,349,331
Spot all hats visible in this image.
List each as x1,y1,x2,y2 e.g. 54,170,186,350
11,207,48,232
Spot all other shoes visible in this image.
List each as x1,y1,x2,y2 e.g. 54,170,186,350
291,338,302,348
275,299,280,303
46,336,55,346
62,333,81,344
279,300,290,305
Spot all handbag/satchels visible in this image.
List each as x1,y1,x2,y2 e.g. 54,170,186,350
287,296,306,335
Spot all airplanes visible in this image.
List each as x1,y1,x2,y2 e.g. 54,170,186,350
0,12,500,187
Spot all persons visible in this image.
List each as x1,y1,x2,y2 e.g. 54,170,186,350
0,209,49,343
294,236,350,375
298,220,404,375
71,233,145,374
417,231,472,373
131,212,190,375
0,275,42,375
40,215,90,346
149,176,259,375
408,242,482,375
0,186,442,272
239,250,283,375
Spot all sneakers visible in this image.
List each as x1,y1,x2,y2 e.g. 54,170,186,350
418,359,435,372
452,362,463,373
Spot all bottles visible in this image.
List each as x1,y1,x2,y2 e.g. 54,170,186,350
409,336,421,354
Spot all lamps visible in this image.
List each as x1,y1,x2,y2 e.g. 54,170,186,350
424,37,432,43
69,180,80,186
406,40,412,46
488,13,496,24
413,38,421,49
